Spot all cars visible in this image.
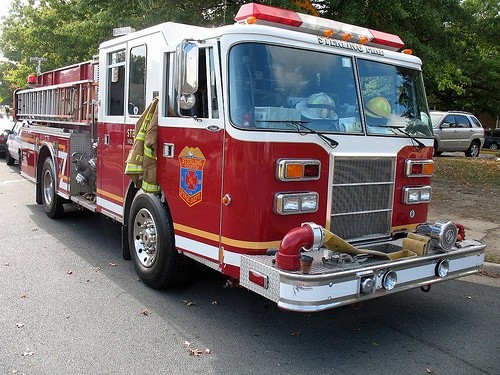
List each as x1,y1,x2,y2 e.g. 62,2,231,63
484,129,499,150
429,110,485,157
6,122,22,165
0,118,16,158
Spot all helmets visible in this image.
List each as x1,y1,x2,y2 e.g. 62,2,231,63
297,92,337,121
364,96,391,119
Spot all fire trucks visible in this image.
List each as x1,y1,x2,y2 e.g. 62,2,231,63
13,2,487,310
